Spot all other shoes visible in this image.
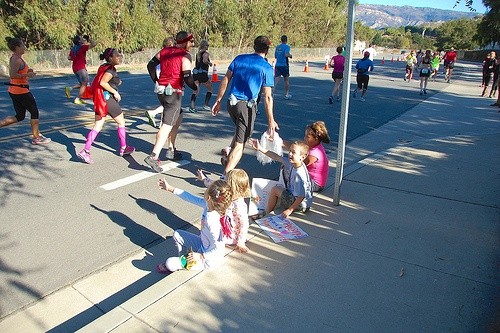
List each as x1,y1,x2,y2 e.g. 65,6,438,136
252,211,265,220
203,105,211,111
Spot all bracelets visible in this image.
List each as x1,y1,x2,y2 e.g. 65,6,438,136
215,100,221,103
196,84,198,88
89,39,92,42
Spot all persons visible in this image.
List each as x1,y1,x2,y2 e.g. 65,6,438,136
65,35,98,106
213,34,279,174
352,51,374,102
195,169,250,254
249,138,312,220
77,47,135,165
272,35,291,100
404,48,426,83
144,31,199,173
428,47,456,83
145,37,175,127
0,36,52,144
419,50,432,95
189,39,213,113
267,121,330,191
340,47,346,85
481,51,500,113
329,46,346,104
156,178,234,274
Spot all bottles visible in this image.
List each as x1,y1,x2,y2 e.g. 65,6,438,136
165,83,173,95
199,67,203,74
104,91,110,100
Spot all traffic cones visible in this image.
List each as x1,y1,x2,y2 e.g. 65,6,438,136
210,64,222,82
392,55,405,62
303,60,310,72
323,59,329,70
81,75,94,100
382,57,384,64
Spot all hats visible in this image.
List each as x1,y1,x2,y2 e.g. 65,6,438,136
364,51,370,56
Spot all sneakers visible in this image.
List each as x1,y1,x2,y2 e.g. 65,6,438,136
221,147,231,167
420,91,423,95
78,149,92,163
285,95,292,99
120,145,135,155
360,98,365,101
353,90,357,98
74,99,85,104
337,98,341,101
144,156,163,173
65,87,70,98
166,150,182,160
329,96,333,104
145,110,156,127
189,107,197,113
156,263,171,274
423,90,427,94
32,134,51,144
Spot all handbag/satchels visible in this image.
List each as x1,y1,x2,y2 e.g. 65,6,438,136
357,68,368,74
